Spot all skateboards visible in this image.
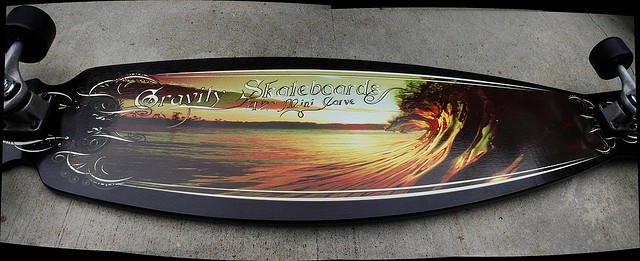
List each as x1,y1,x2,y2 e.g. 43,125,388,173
3,6,637,225
